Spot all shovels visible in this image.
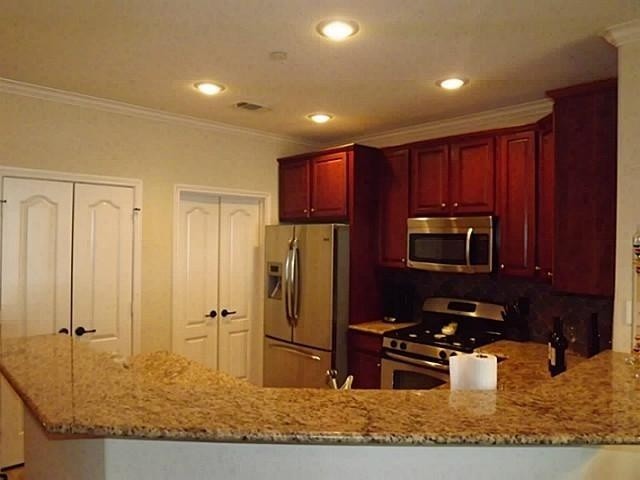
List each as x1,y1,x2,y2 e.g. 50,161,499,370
518,295,529,319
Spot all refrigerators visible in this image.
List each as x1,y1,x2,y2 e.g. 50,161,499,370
264,224,349,388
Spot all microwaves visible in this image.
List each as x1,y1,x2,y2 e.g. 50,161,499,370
406,217,493,273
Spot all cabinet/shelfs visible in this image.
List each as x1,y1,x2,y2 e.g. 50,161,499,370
346,331,383,388
408,128,495,217
544,75,618,297
276,143,373,224
373,143,409,267
495,119,537,277
538,111,552,283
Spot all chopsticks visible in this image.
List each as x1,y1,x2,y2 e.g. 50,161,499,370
501,303,520,321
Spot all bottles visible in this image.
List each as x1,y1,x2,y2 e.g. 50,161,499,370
548,317,564,372
592,312,600,354
550,320,568,377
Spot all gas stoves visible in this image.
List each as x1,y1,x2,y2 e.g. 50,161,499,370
382,321,503,361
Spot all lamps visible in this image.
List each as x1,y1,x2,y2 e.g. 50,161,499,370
193,80,225,95
315,17,360,42
305,112,333,123
435,74,469,91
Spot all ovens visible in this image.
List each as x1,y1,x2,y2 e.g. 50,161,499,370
380,351,449,389
